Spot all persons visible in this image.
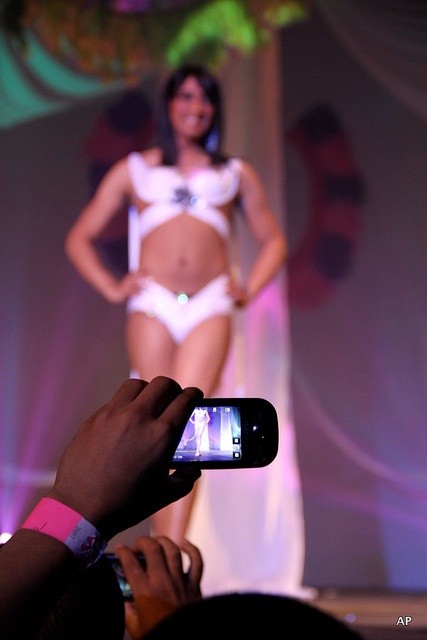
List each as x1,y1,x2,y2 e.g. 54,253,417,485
115,535,204,640
66,63,287,553
189,407,210,456
0,377,204,625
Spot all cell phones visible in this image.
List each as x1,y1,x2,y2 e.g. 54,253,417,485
170,396,279,470
100,552,147,599
